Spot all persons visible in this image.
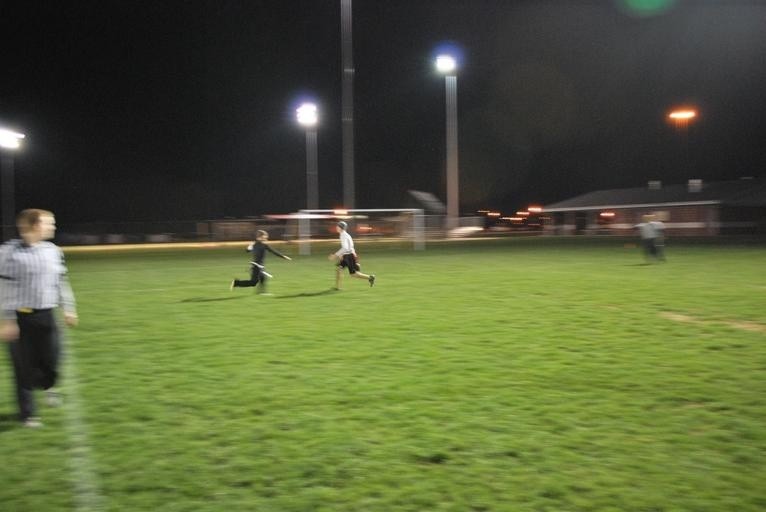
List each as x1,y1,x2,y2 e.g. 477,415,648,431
0,209,80,432
634,212,669,266
230,230,292,297
327,221,375,291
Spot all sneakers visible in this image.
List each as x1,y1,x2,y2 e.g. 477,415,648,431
230,279,236,292
21,383,60,428
368,274,375,287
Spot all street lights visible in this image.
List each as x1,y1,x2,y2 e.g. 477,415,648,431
666,108,697,180
435,51,464,231
296,100,325,216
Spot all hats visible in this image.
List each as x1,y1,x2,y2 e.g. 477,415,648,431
337,221,346,228
